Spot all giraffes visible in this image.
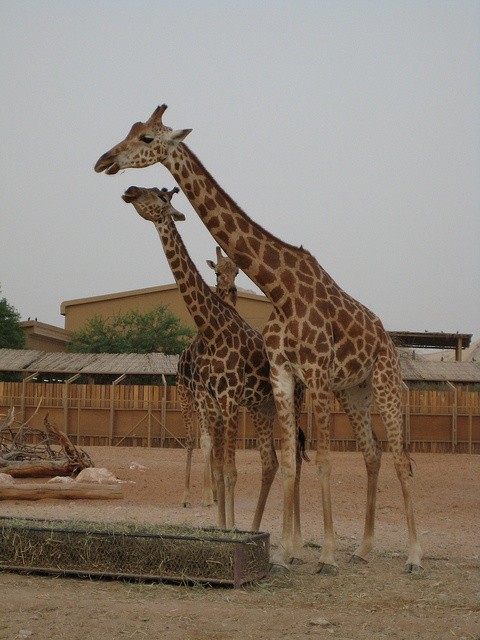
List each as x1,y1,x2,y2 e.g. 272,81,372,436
121,185,311,566
175,246,248,509
93,104,424,576
